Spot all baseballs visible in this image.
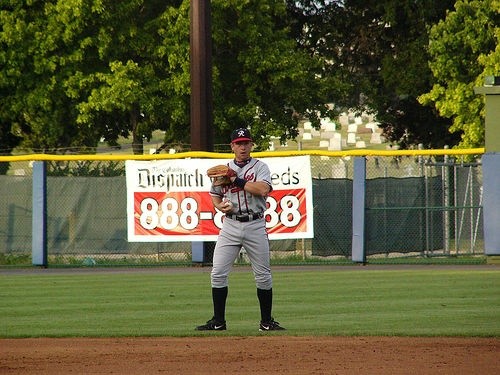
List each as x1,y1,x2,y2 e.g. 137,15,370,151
225,202,233,210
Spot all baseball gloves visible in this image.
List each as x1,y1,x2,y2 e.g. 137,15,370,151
207,165,237,186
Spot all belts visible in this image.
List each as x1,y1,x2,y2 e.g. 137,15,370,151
226,211,263,222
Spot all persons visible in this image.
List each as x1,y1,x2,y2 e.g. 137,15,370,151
195,128,286,331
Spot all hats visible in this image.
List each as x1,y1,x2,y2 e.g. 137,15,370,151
231,128,252,143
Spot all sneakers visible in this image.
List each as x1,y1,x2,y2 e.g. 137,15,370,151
198,316,226,330
259,318,285,331
